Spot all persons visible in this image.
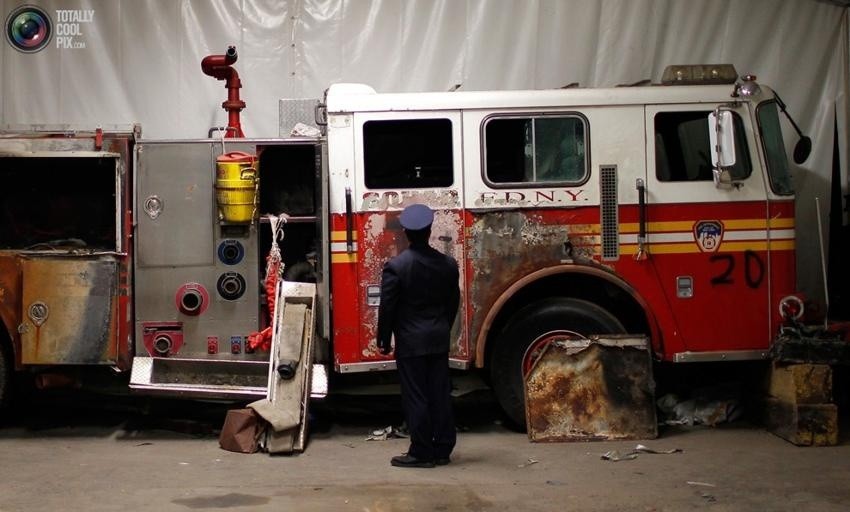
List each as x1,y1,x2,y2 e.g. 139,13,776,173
374,202,463,468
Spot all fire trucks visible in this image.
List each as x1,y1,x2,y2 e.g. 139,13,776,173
1,42,816,435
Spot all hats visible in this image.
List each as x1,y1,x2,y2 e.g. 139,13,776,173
399,204,434,235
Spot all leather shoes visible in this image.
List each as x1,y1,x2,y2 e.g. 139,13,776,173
390,454,436,468
436,457,450,465
